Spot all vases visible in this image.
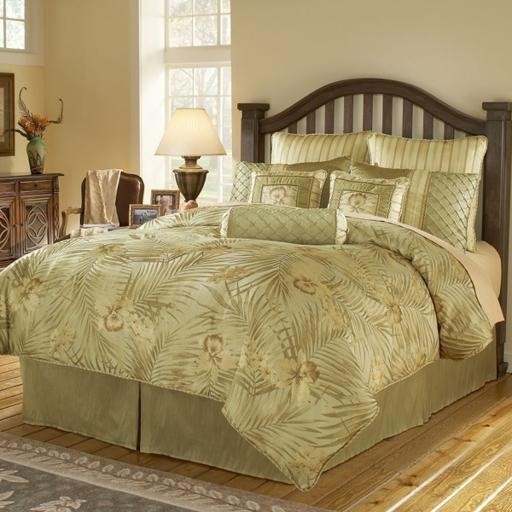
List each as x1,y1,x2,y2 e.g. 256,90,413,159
26,139,47,174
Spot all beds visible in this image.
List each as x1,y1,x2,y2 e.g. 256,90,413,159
0,79,512,492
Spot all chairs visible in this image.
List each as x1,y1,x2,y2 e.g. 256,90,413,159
59,171,144,239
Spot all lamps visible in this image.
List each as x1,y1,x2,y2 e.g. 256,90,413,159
152,108,227,209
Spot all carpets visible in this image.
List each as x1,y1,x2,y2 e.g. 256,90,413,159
0,432,330,512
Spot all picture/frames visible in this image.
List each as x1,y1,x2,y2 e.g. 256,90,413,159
0,73,14,157
152,190,180,213
129,204,160,226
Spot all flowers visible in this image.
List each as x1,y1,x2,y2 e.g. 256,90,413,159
6,84,65,138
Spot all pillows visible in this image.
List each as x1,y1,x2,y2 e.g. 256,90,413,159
344,158,482,254
247,168,333,209
228,156,351,209
270,129,369,167
328,170,409,225
369,132,488,175
222,203,350,249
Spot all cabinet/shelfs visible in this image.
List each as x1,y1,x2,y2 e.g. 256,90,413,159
0,174,62,270
